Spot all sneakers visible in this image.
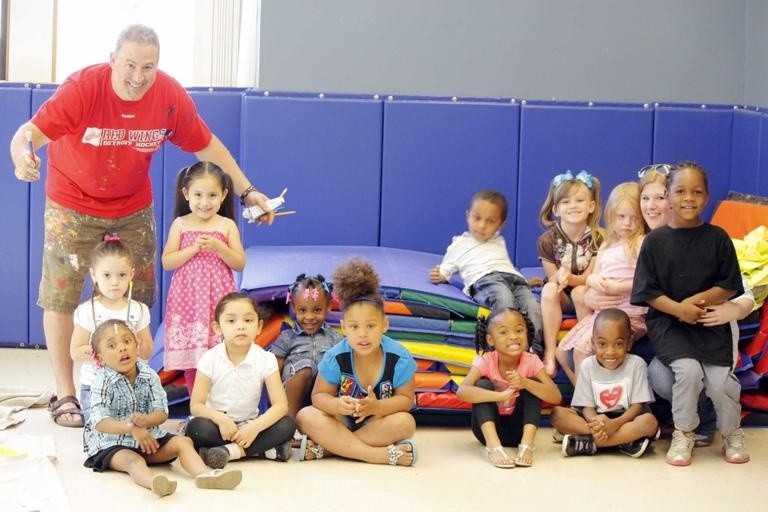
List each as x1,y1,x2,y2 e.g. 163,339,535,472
667,431,697,466
723,430,749,463
694,429,713,446
562,434,597,456
614,437,649,458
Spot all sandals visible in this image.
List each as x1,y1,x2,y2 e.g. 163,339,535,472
553,429,565,441
49,395,84,427
248,443,291,462
197,470,241,489
152,475,176,496
517,445,534,467
199,445,229,469
485,446,515,469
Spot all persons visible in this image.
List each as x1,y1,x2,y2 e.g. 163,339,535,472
630,160,750,465
456,307,562,469
584,164,757,448
84,320,243,495
161,161,246,432
295,257,417,465
10,26,275,427
541,172,605,377
551,309,661,459
184,293,296,469
268,274,345,449
430,190,544,363
551,182,652,442
70,232,154,424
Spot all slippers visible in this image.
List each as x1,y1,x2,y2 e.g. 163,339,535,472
388,439,416,465
300,435,323,461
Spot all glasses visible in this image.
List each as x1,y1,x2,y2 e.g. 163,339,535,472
637,164,675,178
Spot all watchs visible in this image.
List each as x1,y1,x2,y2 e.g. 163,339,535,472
240,183,256,208
